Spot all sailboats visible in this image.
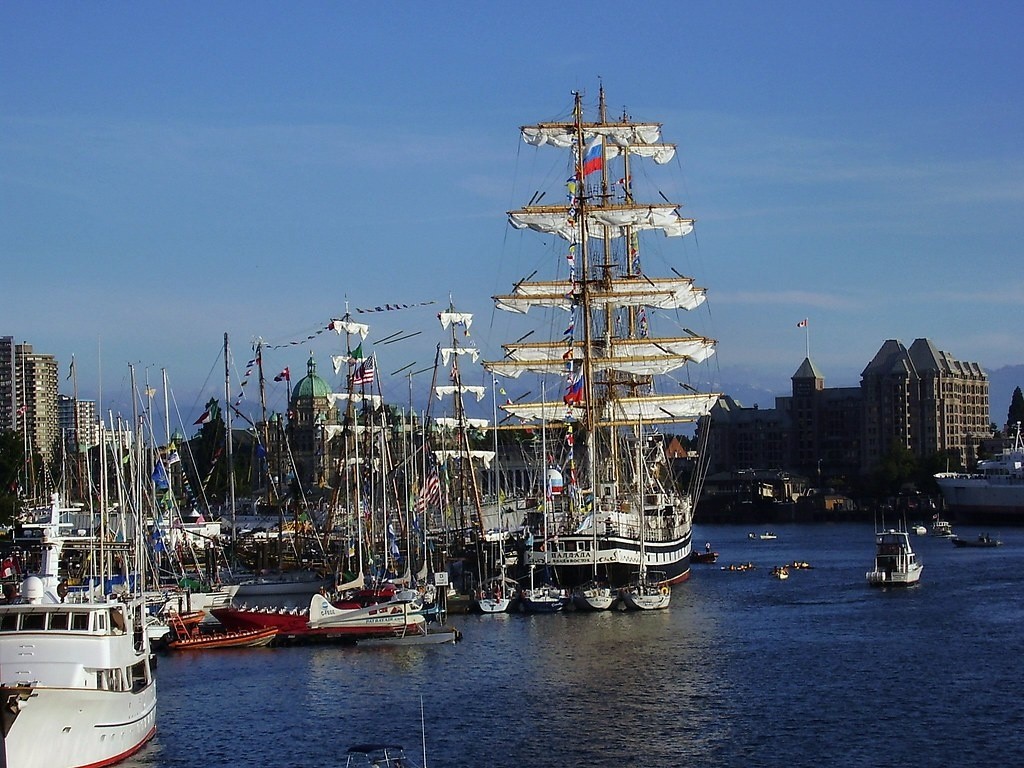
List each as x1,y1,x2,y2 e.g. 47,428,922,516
0,70,723,767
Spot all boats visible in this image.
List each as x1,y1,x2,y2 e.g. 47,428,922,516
866,528,924,591
912,526,927,535
792,562,815,570
930,522,958,540
720,566,755,573
748,533,756,540
772,569,788,580
761,535,777,540
931,420,1024,526
690,549,719,564
951,537,1005,547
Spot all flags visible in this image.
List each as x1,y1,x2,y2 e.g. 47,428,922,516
66,350,265,598
282,375,595,590
1,555,21,577
643,373,657,397
565,132,581,514
582,132,604,176
932,514,938,519
610,174,649,339
450,313,540,441
348,341,375,385
6,404,31,495
547,451,555,462
546,474,553,499
256,301,435,350
272,367,291,383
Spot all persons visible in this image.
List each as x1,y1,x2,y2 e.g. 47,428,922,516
570,515,579,530
721,531,809,575
977,531,992,542
705,540,710,554
605,516,615,537
